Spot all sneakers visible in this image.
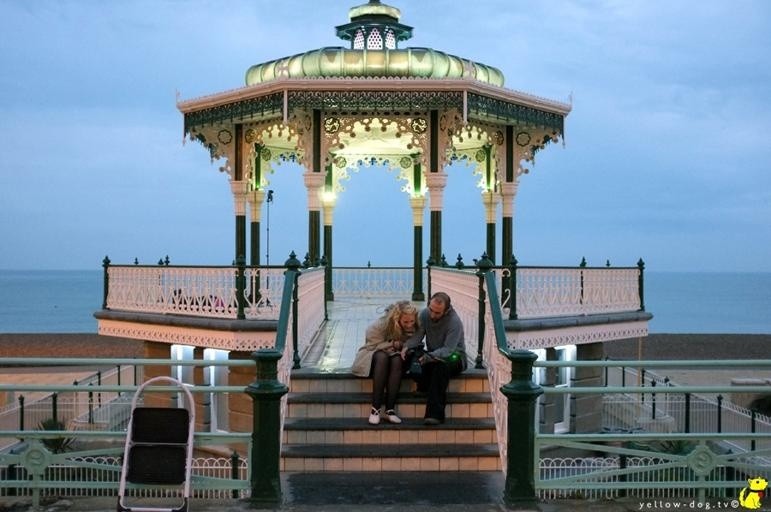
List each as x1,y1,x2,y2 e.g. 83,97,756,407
423,418,440,424
416,383,428,396
384,410,401,423
369,407,381,424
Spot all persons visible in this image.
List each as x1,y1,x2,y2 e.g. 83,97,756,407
349,298,423,426
397,292,469,428
